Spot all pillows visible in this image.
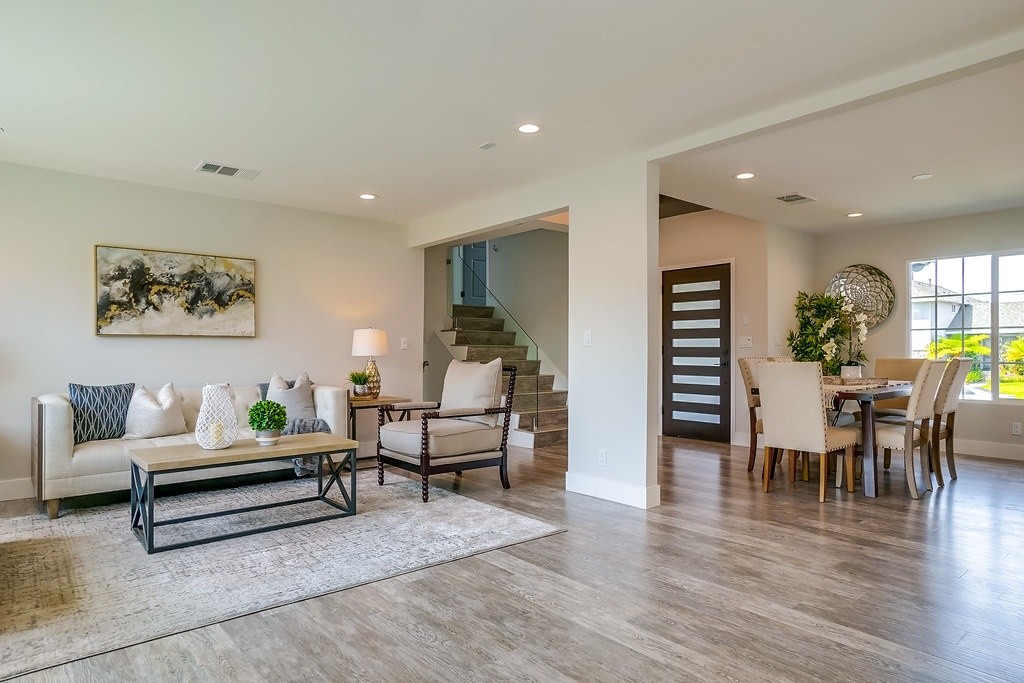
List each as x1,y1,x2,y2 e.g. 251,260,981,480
68,382,135,445
438,358,502,429
267,373,315,419
121,382,189,440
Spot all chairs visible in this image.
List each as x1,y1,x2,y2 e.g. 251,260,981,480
739,356,973,503
377,362,517,503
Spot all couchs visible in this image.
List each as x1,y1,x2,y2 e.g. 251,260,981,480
30,379,350,520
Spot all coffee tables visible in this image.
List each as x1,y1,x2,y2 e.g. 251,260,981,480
129,433,359,554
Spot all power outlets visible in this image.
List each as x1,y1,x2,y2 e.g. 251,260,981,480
1011,421,1022,435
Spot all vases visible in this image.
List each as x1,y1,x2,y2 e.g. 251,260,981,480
841,366,861,378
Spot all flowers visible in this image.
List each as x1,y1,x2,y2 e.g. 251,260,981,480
819,304,870,368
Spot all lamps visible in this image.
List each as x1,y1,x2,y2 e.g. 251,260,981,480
351,327,389,399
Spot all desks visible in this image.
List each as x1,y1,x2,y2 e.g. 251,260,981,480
350,395,412,470
750,380,934,498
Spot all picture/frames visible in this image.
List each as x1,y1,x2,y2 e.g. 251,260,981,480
93,244,256,338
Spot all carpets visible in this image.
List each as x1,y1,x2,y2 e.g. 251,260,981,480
0,468,572,683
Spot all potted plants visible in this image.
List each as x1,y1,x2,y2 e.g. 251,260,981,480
346,370,372,397
248,400,288,438
